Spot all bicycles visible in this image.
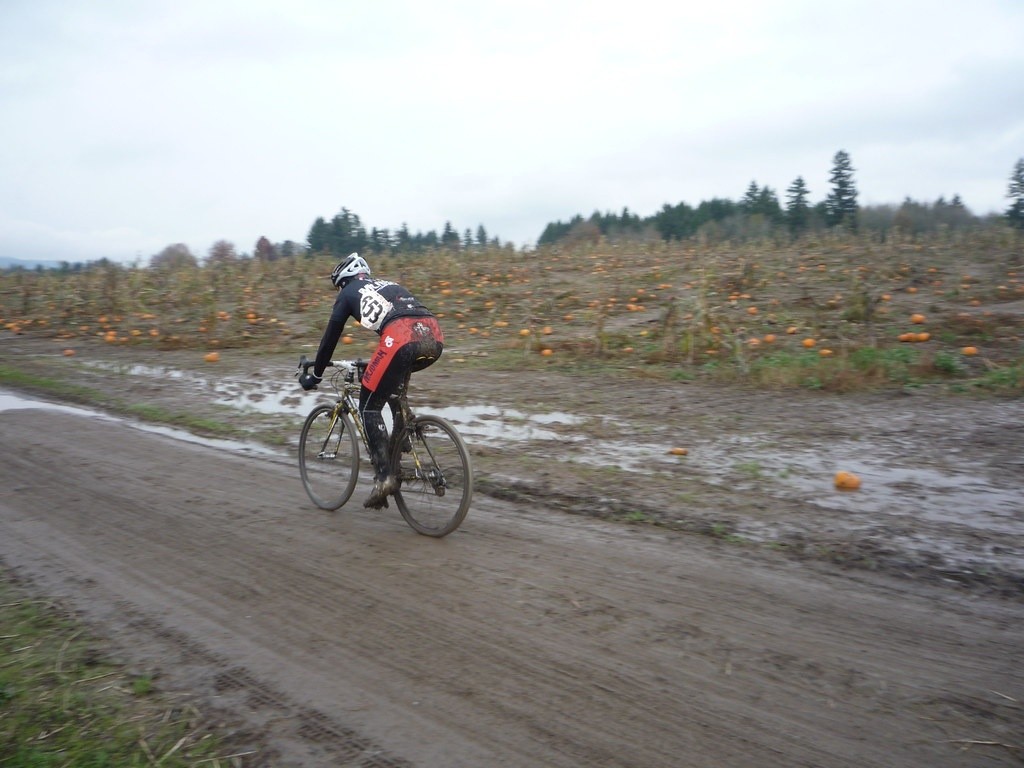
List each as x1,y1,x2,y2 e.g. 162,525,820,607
287,353,474,538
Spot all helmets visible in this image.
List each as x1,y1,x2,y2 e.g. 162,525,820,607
330,252,370,287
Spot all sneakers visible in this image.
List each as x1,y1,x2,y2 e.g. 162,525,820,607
363,476,398,509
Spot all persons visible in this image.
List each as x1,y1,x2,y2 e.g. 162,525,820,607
299,252,443,507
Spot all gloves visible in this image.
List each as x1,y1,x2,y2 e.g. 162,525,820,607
299,372,322,392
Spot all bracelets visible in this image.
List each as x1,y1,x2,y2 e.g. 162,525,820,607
313,372,322,379
